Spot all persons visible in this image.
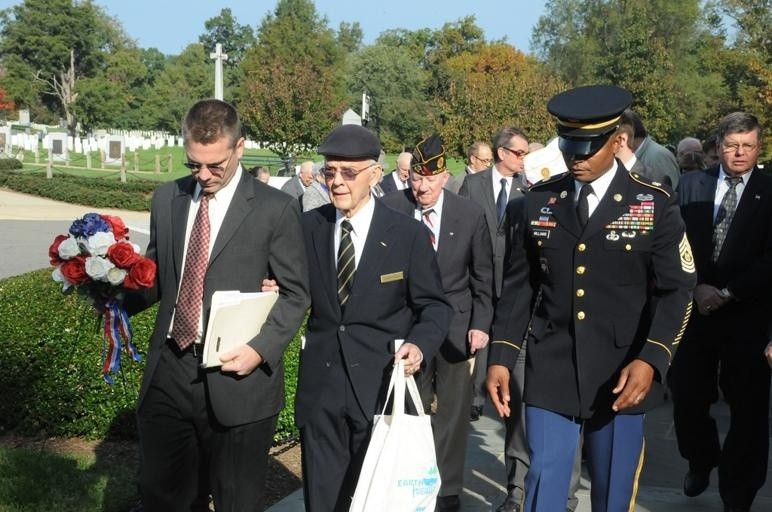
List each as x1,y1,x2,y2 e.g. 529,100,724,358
251,84,771,511
96,99,311,511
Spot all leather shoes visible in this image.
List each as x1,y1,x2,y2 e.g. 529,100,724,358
682,442,722,498
497,497,521,511
467,404,485,421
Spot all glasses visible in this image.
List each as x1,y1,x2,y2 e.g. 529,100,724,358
722,141,758,152
471,154,491,164
318,163,374,182
183,157,230,175
499,145,527,158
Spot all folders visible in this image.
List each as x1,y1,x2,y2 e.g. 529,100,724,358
203,291,281,368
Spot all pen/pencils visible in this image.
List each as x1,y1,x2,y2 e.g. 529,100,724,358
200,362,208,369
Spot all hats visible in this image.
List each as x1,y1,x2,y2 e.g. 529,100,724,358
545,83,633,156
317,124,381,162
410,133,446,177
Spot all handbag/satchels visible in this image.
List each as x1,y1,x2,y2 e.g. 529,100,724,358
348,336,443,512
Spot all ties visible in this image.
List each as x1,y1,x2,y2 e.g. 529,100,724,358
573,183,594,225
709,176,743,262
402,180,408,190
335,219,358,309
495,175,510,224
170,191,215,350
419,208,436,248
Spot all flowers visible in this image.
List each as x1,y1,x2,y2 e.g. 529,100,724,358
46,212,158,332
